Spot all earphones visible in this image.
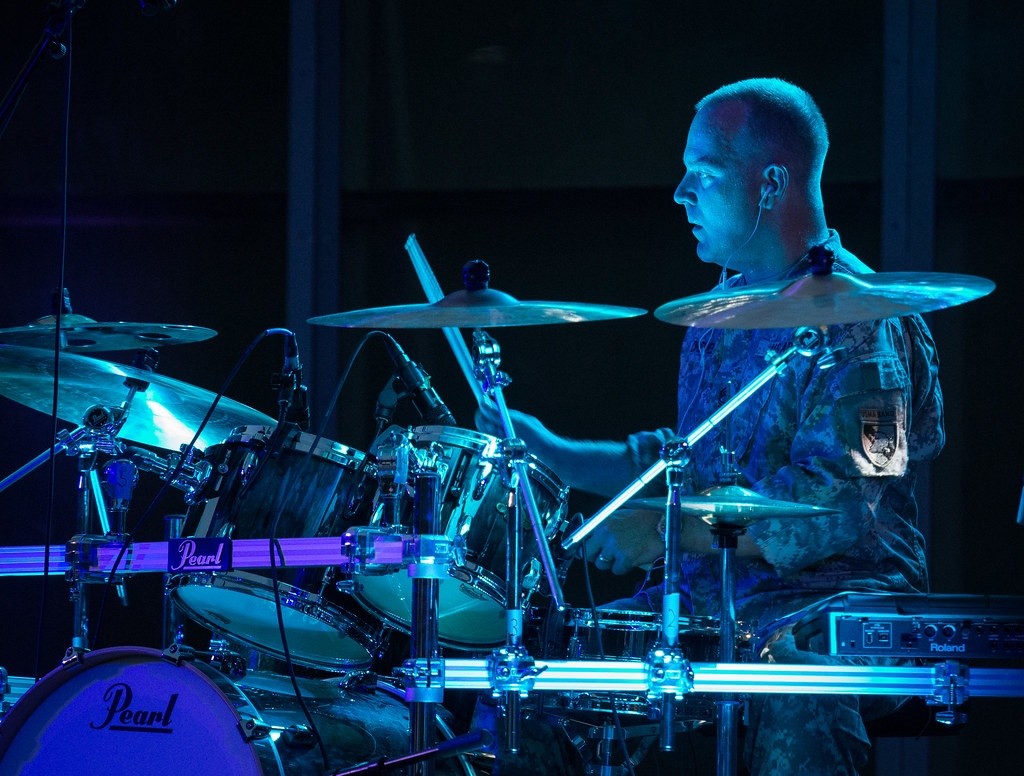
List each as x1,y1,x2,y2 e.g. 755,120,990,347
758,185,775,206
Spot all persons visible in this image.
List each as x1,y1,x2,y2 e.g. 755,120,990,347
471,77,946,776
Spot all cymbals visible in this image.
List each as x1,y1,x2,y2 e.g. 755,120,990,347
0,343,280,455
653,271,997,330
619,484,841,524
0,314,220,350
305,288,649,330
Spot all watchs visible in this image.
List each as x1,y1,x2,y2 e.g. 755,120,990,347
655,513,683,541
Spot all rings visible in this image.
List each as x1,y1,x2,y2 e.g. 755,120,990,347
598,553,611,563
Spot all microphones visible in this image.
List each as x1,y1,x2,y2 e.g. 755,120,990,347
280,333,310,431
270,373,280,400
382,334,457,427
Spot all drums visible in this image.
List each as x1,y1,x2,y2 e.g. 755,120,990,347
338,416,571,653
168,422,395,675
517,604,756,723
0,643,477,775
